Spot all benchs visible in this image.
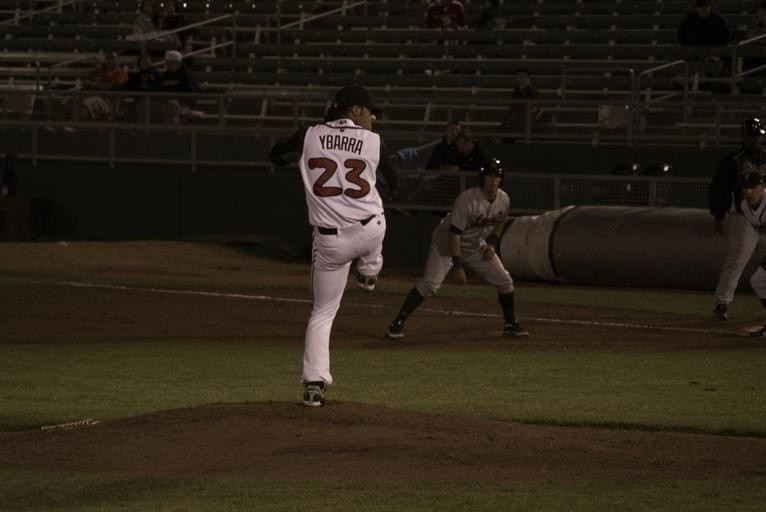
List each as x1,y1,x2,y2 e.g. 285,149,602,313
0,0,762,111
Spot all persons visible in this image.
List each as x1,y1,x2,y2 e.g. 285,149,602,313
388,157,529,339
672,0,766,93
496,69,538,144
79,0,200,124
708,117,766,337
271,86,387,407
391,120,493,217
425,0,505,76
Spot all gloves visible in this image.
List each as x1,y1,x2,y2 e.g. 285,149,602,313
714,217,726,233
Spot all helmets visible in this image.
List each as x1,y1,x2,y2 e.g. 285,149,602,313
743,118,766,135
479,158,504,178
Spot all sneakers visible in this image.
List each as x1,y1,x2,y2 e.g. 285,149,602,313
712,304,728,320
750,327,765,336
386,324,404,338
302,384,325,406
503,322,529,337
356,275,376,290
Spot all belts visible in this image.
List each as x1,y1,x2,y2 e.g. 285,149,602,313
318,215,375,235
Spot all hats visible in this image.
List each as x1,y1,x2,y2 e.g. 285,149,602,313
742,172,760,188
331,86,383,114
